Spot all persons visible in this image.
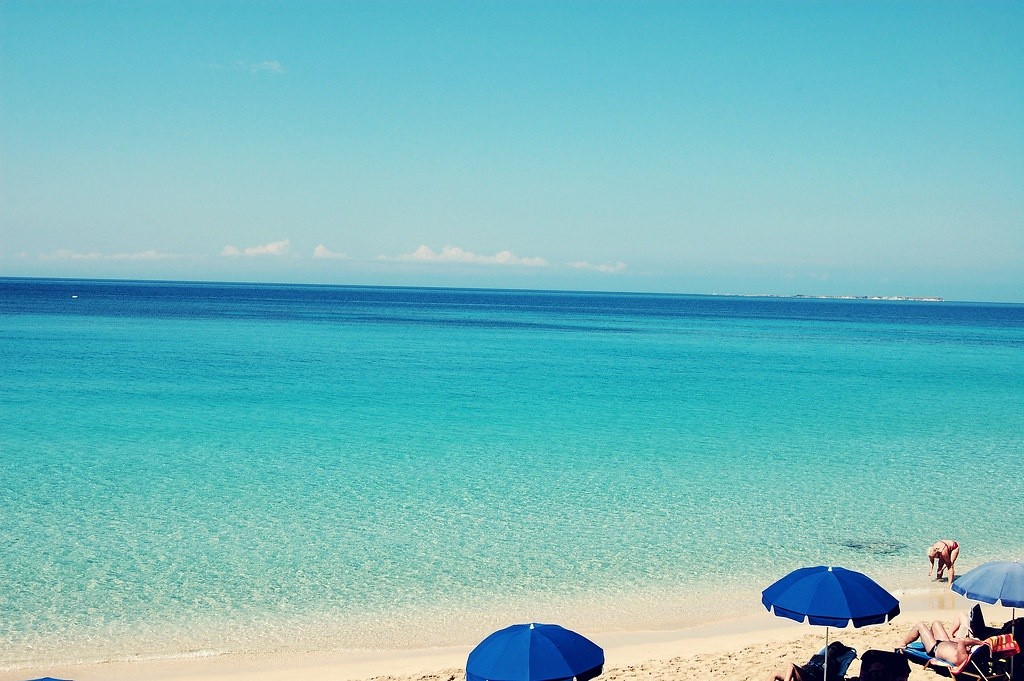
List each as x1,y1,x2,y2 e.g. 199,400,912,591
773,641,847,681
892,616,993,667
927,540,960,586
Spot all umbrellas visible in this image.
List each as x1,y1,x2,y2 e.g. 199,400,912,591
466,623,605,681
950,559,1024,681
762,566,900,681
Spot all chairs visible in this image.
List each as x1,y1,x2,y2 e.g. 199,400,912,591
859,650,911,681
894,634,1020,681
818,645,858,681
